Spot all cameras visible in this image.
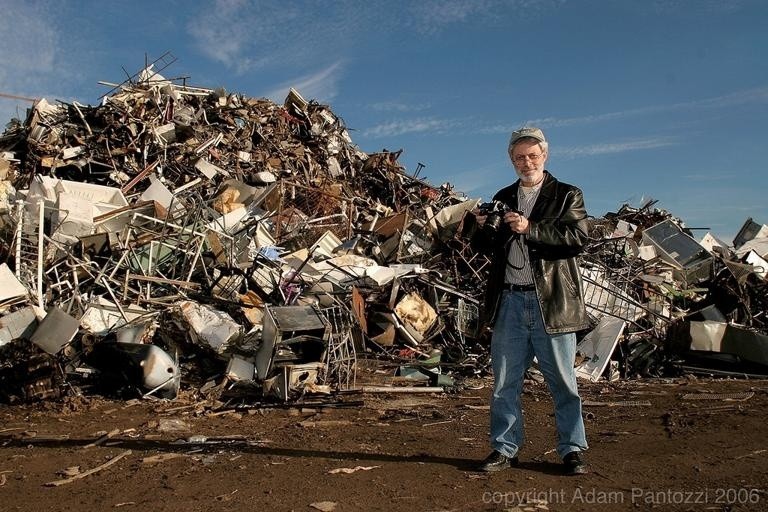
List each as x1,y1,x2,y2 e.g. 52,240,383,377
479,202,524,235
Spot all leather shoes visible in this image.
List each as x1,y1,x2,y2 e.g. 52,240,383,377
563,450,591,475
478,448,521,472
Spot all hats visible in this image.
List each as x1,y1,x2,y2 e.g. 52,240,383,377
509,127,544,146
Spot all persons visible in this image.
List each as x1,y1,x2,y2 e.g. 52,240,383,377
470,127,590,476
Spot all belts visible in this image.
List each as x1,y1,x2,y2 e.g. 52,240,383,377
500,282,539,292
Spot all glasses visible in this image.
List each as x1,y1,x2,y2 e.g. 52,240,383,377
509,151,545,161
